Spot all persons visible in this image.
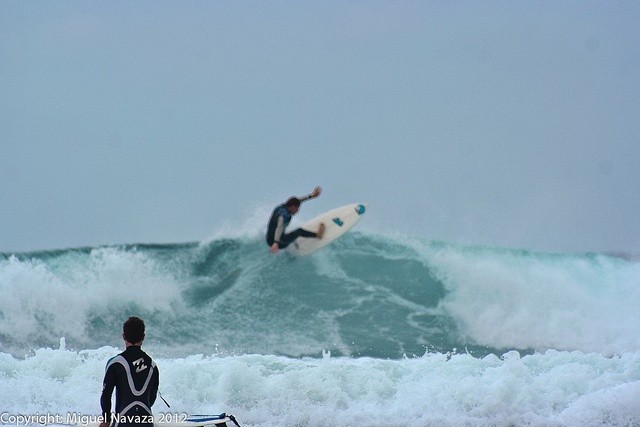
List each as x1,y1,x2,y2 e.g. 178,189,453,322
99,316,160,426
264,186,326,253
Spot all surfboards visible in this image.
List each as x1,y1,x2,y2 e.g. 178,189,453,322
285,202,368,256
16,411,235,427
